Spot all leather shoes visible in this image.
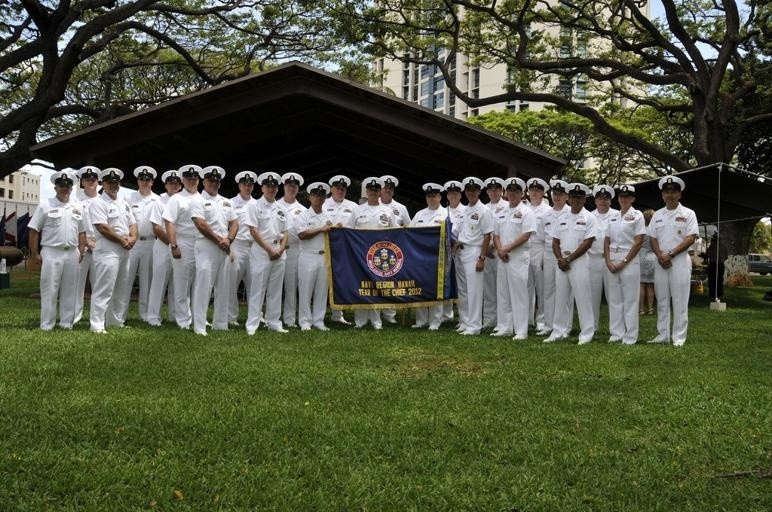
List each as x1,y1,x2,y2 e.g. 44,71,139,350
411,324,684,347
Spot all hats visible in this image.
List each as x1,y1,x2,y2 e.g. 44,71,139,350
422,176,685,196
234,170,305,186
161,165,226,183
329,174,351,187
307,181,331,196
133,165,156,180
361,174,400,191
50,165,125,181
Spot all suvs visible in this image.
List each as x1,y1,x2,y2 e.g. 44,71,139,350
747,253,772,276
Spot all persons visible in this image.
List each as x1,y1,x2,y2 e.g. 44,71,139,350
699,230,728,301
26,162,700,348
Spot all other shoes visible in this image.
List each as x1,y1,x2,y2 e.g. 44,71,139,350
138,314,241,336
244,312,397,337
92,325,130,337
